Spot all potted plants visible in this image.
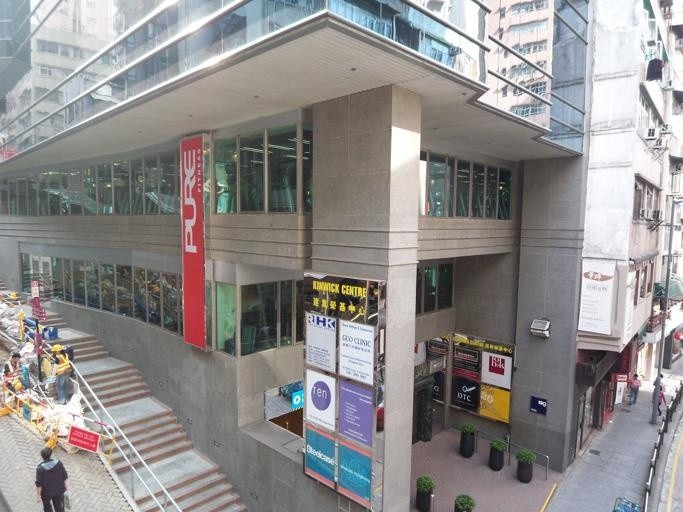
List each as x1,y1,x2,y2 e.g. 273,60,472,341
459,422,536,483
415,473,475,511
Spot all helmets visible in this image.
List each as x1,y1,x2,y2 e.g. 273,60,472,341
50,344,61,351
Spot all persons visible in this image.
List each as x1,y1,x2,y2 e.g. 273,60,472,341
3,351,30,395
34,446,69,512
653,373,665,392
652,386,667,416
627,373,642,405
51,343,71,404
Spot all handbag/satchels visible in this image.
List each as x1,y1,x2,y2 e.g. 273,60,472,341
63,491,72,511
